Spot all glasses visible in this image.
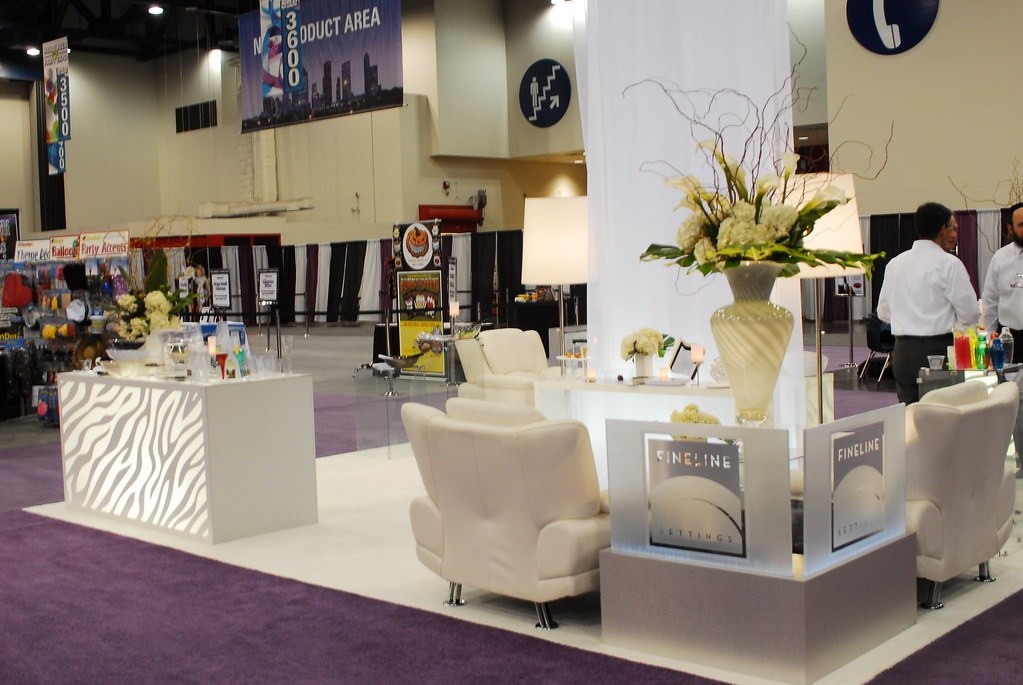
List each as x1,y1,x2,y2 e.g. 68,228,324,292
945,224,953,230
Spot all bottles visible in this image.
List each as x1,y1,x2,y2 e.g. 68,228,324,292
160,319,246,384
954,315,1014,370
531,287,560,302
73,316,114,370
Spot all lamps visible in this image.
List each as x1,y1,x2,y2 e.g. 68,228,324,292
449,302,460,339
522,196,587,379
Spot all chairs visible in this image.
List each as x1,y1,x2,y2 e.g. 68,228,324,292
352,352,422,397
857,325,894,389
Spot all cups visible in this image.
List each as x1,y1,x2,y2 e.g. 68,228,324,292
928,356,945,369
947,346,956,370
80,360,92,373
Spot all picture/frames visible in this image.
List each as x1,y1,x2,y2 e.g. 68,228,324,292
0,207,21,260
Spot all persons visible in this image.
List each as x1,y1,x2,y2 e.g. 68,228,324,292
980,202,1023,364
189,265,210,323
877,202,979,406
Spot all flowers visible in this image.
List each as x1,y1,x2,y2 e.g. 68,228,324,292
670,403,718,440
624,23,884,281
622,326,674,359
100,245,204,338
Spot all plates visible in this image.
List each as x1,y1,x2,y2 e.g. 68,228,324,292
645,378,688,386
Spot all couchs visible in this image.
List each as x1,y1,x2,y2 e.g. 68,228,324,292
897,379,1021,608
397,396,607,631
455,330,594,410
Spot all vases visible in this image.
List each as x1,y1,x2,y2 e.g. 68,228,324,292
110,339,146,351
710,260,795,421
633,353,653,378
